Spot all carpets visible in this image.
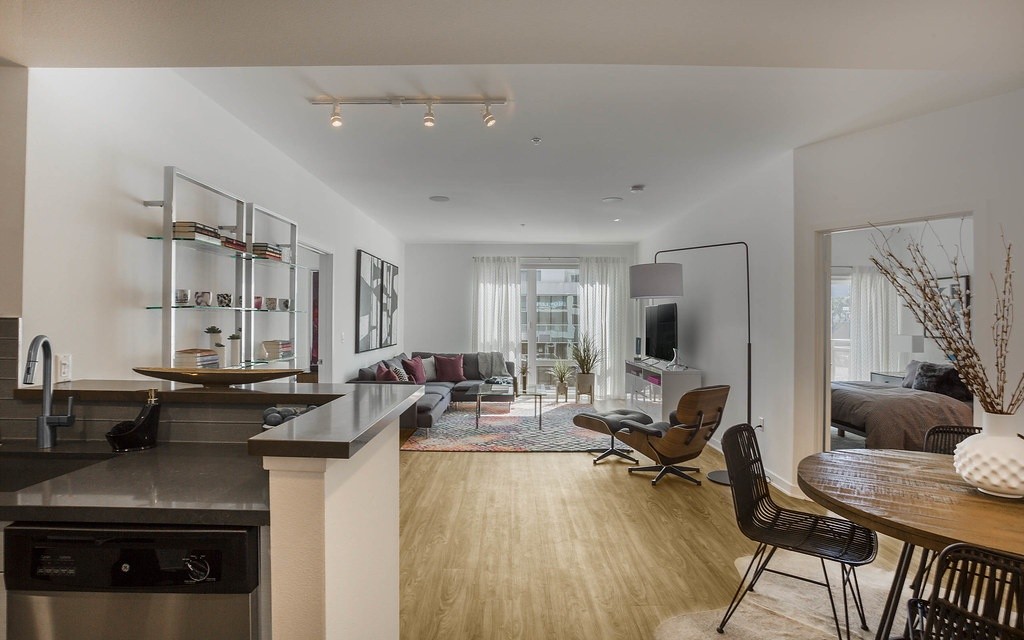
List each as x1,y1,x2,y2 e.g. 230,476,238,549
655,551,1016,640
400,401,630,452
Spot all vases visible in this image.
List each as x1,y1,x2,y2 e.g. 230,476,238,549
953,411,1024,499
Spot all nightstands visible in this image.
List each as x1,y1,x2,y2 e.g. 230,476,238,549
871,372,905,385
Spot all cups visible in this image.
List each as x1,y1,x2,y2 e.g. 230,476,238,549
216,294,233,308
194,291,212,307
238,296,243,306
265,298,278,311
278,299,289,311
254,295,262,309
174,289,191,305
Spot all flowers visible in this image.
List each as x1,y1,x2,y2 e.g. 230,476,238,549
866,215,1024,415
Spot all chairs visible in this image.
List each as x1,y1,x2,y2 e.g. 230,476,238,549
615,384,731,486
720,422,1024,640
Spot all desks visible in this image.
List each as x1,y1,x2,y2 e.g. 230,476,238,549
797,449,1024,639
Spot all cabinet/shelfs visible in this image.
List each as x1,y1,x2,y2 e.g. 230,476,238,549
144,166,298,383
625,360,702,423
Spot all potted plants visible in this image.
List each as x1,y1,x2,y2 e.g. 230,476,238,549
204,326,222,354
227,334,241,366
215,343,226,368
546,331,605,394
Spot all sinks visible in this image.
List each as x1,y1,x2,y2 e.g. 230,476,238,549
0,451,125,492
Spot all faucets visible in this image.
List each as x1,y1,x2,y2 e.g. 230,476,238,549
23,334,75,449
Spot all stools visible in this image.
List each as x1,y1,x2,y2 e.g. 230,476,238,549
574,408,653,465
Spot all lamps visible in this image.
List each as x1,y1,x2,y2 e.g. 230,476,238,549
308,99,507,127
629,242,752,486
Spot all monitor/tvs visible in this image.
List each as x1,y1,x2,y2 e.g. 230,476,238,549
641,302,688,370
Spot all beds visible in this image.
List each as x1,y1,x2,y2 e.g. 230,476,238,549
830,381,973,451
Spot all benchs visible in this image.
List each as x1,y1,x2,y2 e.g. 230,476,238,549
412,352,514,413
345,352,451,438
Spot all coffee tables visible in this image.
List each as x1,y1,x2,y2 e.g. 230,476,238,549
465,385,547,430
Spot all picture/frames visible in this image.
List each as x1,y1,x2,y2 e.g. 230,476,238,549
356,249,399,353
924,275,969,339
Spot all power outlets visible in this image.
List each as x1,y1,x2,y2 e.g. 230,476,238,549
759,417,764,431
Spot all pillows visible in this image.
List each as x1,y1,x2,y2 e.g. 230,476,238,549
901,359,972,402
377,355,466,384
